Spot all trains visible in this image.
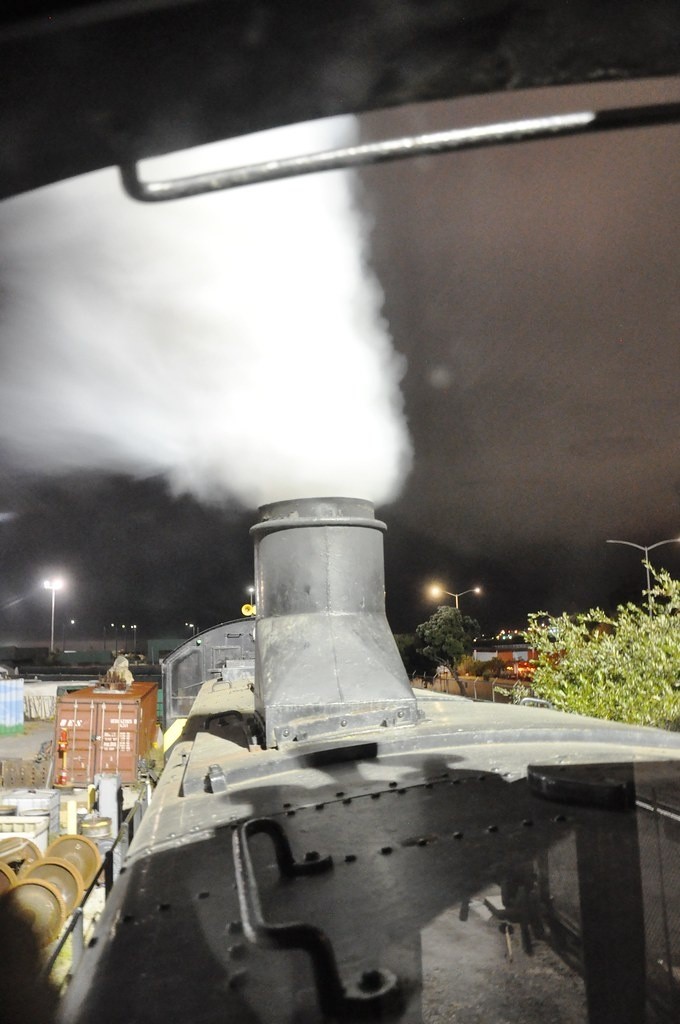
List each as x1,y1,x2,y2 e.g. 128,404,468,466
64,496,680,1024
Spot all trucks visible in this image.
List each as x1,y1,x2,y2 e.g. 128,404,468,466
52,681,159,791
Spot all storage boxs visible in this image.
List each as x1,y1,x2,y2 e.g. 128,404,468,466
54,680,158,784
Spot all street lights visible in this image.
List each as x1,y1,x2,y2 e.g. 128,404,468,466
61,620,75,653
604,536,680,622
112,623,138,657
426,582,483,613
248,587,255,609
43,577,61,652
